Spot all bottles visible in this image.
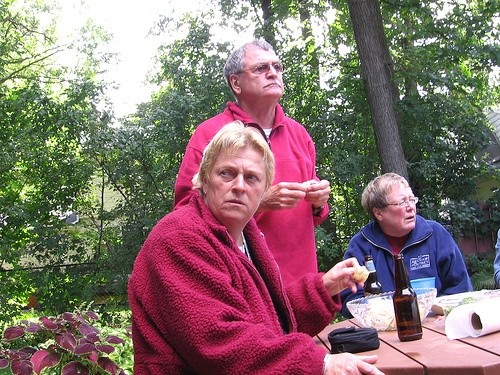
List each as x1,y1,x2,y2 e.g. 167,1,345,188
393,254,423,342
363,255,383,297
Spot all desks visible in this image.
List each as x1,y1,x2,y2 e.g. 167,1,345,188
310,308,500,375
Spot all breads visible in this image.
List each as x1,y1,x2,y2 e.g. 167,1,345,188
353,265,369,282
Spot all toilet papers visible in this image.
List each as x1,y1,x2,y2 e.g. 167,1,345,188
444,296,500,341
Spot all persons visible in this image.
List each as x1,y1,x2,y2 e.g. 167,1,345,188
339,172,472,318
128,122,385,374
175,38,331,287
493,228,500,285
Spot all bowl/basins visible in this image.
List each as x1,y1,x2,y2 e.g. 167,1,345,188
346,287,436,331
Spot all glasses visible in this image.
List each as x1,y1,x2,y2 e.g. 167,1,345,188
235,63,284,75
381,196,419,207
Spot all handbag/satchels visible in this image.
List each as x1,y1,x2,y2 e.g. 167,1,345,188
327,325,380,354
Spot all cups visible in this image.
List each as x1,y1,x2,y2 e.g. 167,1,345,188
409,277,436,317
